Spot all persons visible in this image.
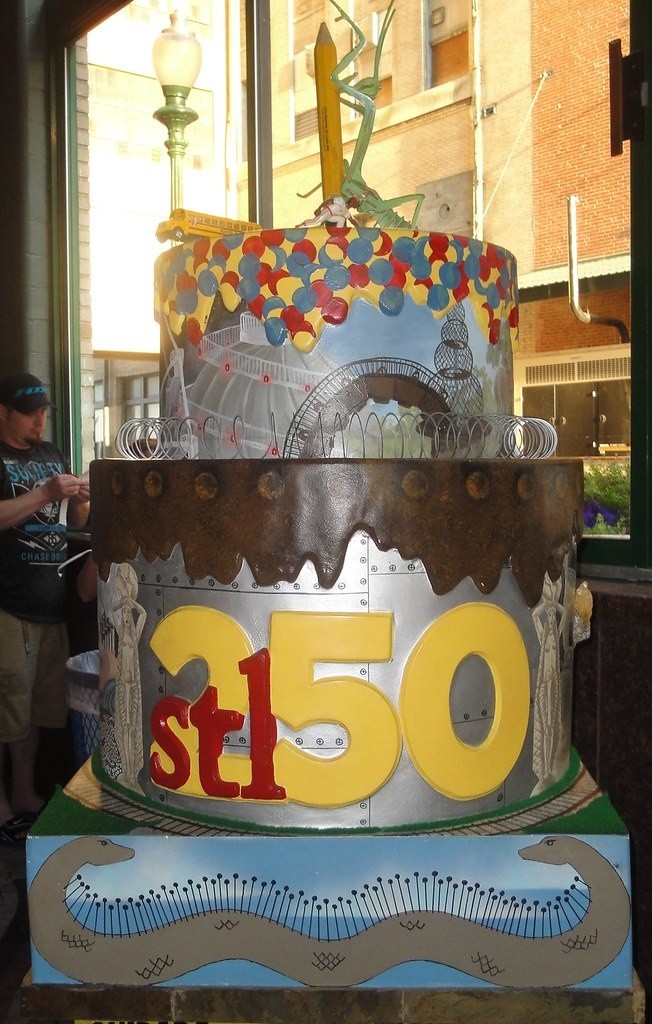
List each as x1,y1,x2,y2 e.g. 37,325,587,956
295,195,360,229
0,372,98,847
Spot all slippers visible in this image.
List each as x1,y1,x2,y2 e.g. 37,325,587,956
13,798,49,815
0,816,33,845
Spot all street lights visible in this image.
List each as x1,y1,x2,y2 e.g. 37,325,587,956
151,11,202,418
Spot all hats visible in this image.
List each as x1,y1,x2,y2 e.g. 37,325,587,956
0,373,58,413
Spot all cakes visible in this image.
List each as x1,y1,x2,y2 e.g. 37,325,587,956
86,222,588,835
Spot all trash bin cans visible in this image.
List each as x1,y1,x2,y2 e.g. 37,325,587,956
64,649,101,770
